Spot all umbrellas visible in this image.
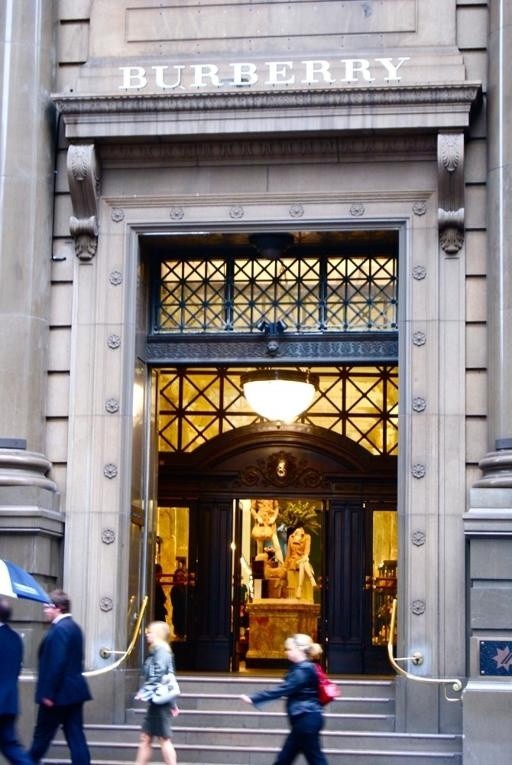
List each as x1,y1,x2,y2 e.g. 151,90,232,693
0,558,53,607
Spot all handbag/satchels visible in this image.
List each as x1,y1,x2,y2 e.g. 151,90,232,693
150,672,181,705
312,662,341,707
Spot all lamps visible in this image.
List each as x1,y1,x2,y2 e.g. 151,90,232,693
240,370,319,425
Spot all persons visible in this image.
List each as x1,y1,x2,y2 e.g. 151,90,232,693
284,525,318,599
249,499,283,566
240,634,343,765
29,589,94,765
132,622,184,765
1,600,39,764
155,563,167,622
170,572,186,637
